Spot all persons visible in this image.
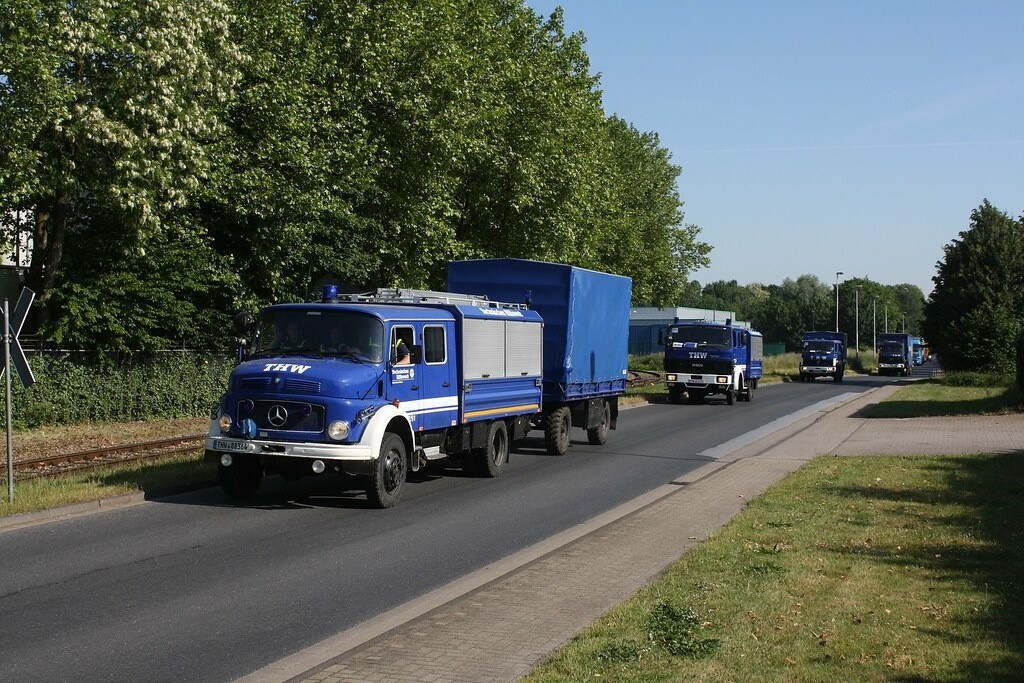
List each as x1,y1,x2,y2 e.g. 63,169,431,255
266,321,312,352
317,326,351,354
347,324,411,366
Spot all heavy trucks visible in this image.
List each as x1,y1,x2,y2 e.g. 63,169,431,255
202,257,633,510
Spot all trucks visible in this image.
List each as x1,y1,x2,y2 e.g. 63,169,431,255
913,334,932,366
877,333,914,377
799,330,848,383
657,317,764,405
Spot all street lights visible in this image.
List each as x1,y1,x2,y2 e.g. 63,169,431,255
884,302,891,334
902,312,908,333
873,295,880,355
835,271,844,334
856,285,864,358
